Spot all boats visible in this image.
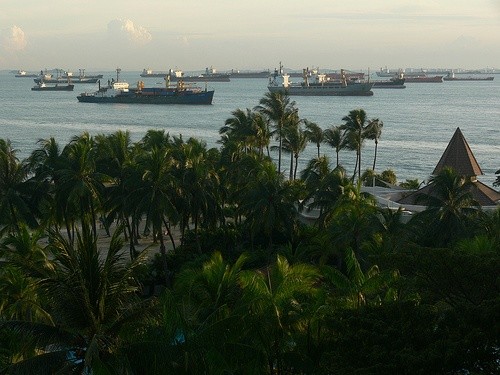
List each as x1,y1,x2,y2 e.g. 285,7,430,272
267,60,407,96
139,68,270,82
76,66,215,105
374,65,494,83
13,68,104,92
